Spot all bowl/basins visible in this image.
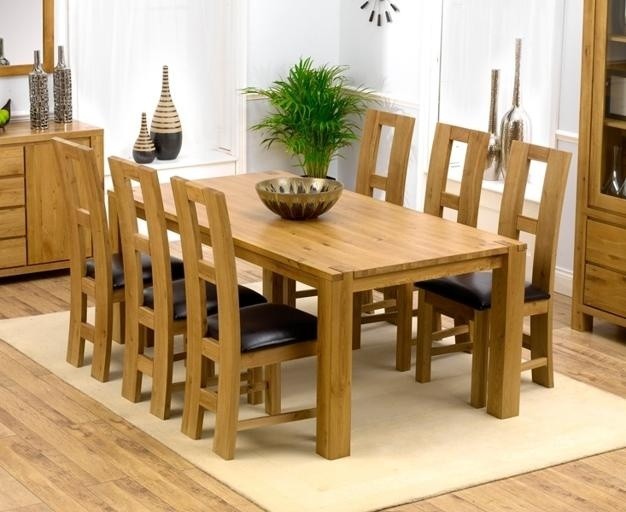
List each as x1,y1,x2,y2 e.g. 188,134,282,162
254,176,344,220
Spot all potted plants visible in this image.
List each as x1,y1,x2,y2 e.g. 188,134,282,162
238,53,382,181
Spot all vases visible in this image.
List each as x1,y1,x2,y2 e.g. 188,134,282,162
0,38,10,64
476,39,532,184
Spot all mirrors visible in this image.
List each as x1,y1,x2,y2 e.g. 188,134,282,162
0,0,55,76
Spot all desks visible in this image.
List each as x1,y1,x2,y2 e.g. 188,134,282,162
107,169,527,460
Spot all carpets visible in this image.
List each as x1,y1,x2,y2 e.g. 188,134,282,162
0,273,626,512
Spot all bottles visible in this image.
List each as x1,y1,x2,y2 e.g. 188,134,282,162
481,69,500,182
501,38,530,187
602,144,626,198
132,111,157,163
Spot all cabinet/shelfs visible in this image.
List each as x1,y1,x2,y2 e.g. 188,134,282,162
0,114,105,280
571,0,626,331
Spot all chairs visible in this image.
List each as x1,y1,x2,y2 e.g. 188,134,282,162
414,139,572,410
171,176,318,461
53,136,184,384
107,155,268,421
352,122,491,372
283,108,417,350
